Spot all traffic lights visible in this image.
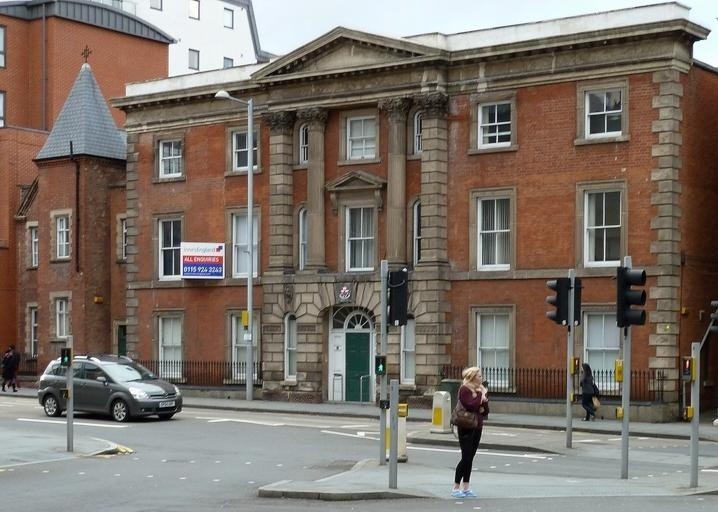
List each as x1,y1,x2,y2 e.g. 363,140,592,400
61,348,72,366
375,356,385,376
545,277,573,327
682,356,696,382
616,265,647,338
570,355,580,375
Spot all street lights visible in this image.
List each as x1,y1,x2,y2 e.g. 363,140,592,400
214,88,254,402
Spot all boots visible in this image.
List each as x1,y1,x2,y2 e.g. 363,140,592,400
2,384,6,392
12,383,18,392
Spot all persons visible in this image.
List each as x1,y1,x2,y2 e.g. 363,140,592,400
579,362,598,421
450,365,490,499
7,344,22,388
1,350,18,392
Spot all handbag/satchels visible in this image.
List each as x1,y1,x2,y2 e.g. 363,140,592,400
593,383,599,395
450,401,478,427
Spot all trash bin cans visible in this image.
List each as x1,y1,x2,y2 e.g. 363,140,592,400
441,379,463,414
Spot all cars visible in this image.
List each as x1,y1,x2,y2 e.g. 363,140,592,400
35,352,182,423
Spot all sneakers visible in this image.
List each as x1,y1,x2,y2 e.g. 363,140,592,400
451,487,477,497
